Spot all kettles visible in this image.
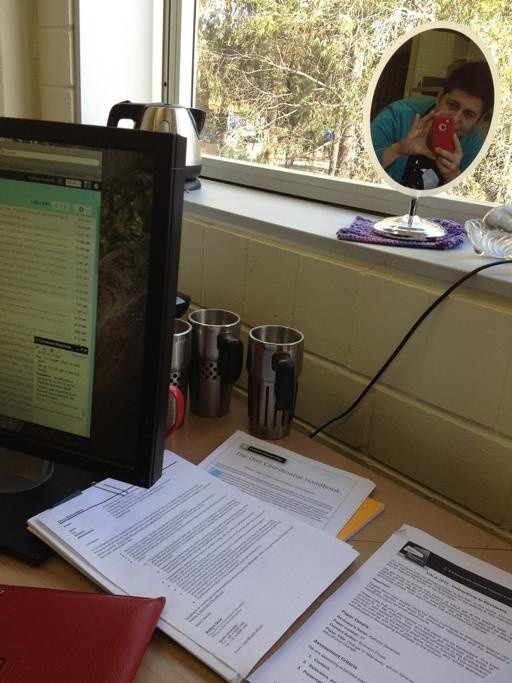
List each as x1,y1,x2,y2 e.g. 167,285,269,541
105,96,208,193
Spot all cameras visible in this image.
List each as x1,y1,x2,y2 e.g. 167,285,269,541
431,113,454,155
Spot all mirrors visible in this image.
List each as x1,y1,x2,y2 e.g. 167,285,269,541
362,20,500,241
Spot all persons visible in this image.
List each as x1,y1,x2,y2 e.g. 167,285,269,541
370,60,494,190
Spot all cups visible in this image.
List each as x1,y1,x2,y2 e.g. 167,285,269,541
245,324,308,443
166,383,185,441
186,309,247,420
165,318,193,427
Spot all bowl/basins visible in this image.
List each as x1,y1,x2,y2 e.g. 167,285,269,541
464,218,512,259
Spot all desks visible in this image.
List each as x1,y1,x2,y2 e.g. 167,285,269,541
0,385,512,683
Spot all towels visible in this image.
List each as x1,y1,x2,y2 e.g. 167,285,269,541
334,213,469,249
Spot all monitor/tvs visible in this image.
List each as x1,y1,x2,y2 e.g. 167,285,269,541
1,114,188,567
